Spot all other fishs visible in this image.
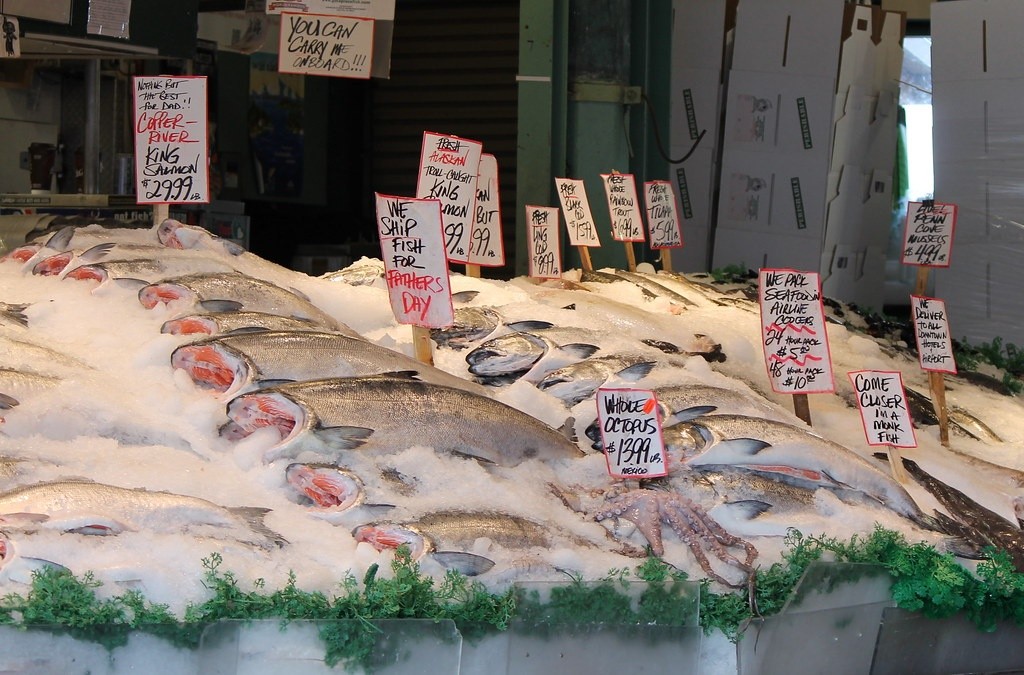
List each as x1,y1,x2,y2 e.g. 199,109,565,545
0,215,1024,586
